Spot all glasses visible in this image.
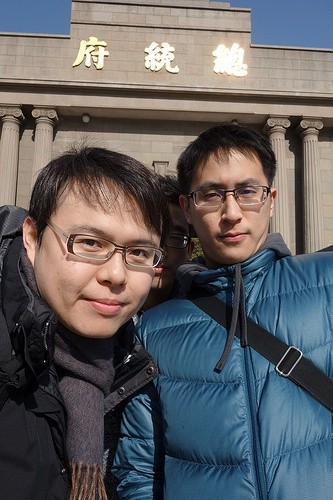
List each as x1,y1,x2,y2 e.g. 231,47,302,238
162,233,191,249
186,186,271,207
44,218,168,268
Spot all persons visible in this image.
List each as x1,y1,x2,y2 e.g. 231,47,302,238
109,125,333,500
131,174,195,326
0,148,173,500
